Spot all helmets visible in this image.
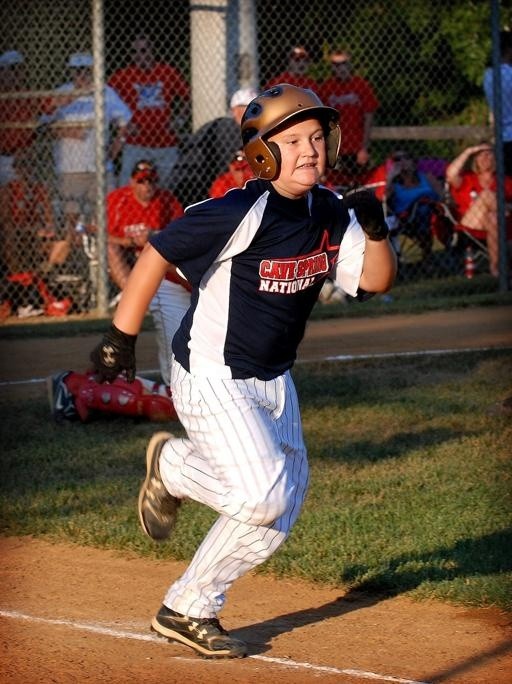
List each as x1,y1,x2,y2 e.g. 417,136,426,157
242,84,342,180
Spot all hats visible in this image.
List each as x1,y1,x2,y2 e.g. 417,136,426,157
132,160,157,180
290,48,309,58
66,52,94,67
1,51,26,67
230,89,259,106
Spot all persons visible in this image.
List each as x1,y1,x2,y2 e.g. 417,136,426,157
1,44,380,313
91,84,400,659
383,139,511,277
484,50,511,177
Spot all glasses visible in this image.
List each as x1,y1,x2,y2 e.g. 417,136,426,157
393,154,411,161
131,48,147,53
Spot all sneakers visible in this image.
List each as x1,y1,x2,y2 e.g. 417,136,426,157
138,432,180,541
47,370,78,429
151,604,248,660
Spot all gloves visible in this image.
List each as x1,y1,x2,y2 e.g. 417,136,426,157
343,183,388,241
89,322,137,386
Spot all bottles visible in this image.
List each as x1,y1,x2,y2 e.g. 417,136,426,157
465,247,475,279
